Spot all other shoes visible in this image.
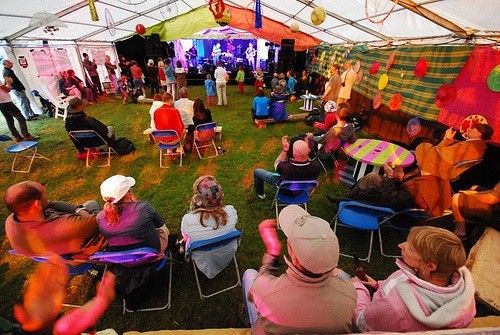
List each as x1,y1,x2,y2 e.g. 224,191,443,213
26,117,38,121
32,114,39,117
16,136,23,143
325,194,349,205
238,302,251,328
23,135,40,141
455,234,467,241
252,184,266,199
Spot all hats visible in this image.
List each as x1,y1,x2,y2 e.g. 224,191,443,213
193,175,223,208
99,175,136,205
67,96,88,113
415,143,460,179
324,101,337,112
279,205,339,274
474,123,494,140
274,86,283,94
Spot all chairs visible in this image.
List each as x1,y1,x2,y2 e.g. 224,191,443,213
0,134,52,173
188,230,243,299
330,201,396,263
151,130,186,168
192,122,218,159
270,181,319,231
313,127,359,173
8,249,107,308
378,209,453,258
32,88,75,121
69,130,118,167
89,247,190,314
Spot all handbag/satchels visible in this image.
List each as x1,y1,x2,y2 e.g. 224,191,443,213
305,109,319,127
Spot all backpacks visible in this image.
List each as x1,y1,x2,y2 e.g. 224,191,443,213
115,136,135,155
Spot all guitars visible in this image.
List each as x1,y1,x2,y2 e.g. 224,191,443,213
246,49,259,59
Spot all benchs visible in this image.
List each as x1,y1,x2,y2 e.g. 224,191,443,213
143,126,223,144
137,98,154,103
255,113,309,128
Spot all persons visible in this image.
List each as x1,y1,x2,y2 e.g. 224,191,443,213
65,97,114,150
328,122,500,238
269,85,288,121
270,70,308,96
251,86,269,126
246,43,256,70
253,67,264,95
253,133,321,199
0,59,39,142
204,60,230,107
57,52,186,104
213,43,221,65
4,174,177,335
308,100,355,160
175,175,239,278
240,204,357,335
318,61,358,123
235,66,245,93
150,87,214,160
352,226,475,333
188,46,197,67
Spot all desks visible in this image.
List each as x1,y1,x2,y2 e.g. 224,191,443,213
299,95,319,112
338,139,415,186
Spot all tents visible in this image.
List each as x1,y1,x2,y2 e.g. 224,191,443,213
0,0,500,152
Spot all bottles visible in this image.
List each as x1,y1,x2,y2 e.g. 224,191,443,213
354,252,368,282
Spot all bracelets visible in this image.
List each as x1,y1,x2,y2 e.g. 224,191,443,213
282,148,289,152
